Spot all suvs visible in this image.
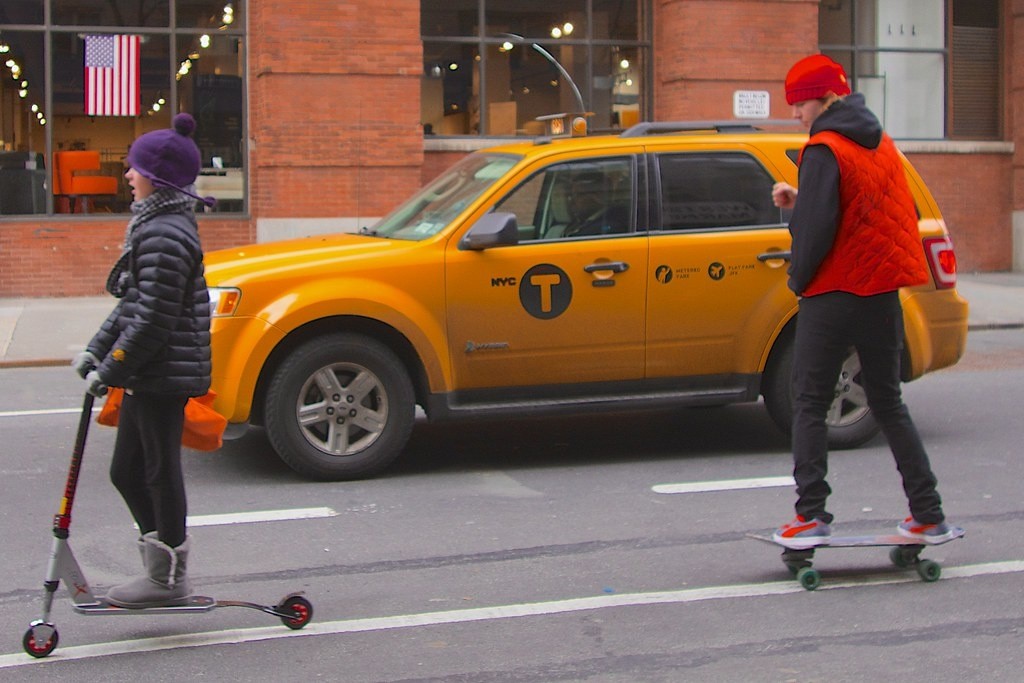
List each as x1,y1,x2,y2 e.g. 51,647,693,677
205,116,969,480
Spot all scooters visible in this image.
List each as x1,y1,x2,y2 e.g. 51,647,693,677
22,364,315,659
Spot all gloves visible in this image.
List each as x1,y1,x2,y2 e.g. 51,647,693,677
72,351,100,379
84,371,108,397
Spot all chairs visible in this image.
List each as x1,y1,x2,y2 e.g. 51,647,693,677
543,182,590,240
52,150,118,213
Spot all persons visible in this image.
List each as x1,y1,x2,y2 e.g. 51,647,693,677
73,113,214,610
769,56,954,545
559,171,625,238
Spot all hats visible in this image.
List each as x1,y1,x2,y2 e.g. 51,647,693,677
783,52,847,105
126,112,216,207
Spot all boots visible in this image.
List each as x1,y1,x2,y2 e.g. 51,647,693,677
105,531,192,609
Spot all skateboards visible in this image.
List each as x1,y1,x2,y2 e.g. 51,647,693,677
745,526,966,589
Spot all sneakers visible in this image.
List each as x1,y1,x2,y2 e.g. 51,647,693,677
896,515,953,543
773,514,833,546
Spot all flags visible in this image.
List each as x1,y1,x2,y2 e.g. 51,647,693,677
82,34,141,117
